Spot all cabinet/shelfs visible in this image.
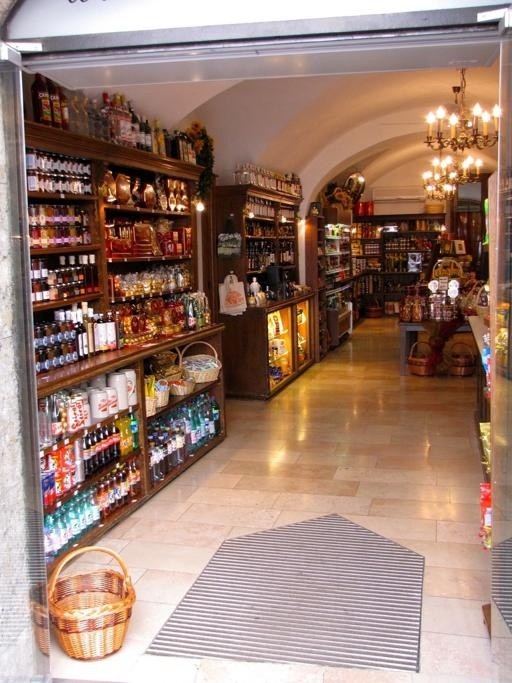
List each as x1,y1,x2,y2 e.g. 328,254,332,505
354,211,447,314
214,183,313,399
23,117,228,575
315,212,355,356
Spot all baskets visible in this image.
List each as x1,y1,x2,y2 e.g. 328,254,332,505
363,297,384,318
47,545,136,660
144,341,222,417
407,341,436,376
424,203,444,214
29,580,47,655
431,259,464,289
447,341,475,376
460,279,488,320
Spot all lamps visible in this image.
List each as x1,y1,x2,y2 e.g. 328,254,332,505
420,65,501,204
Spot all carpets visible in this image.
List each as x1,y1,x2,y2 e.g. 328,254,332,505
144,512,427,672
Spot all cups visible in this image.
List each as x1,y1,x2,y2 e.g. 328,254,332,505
107,366,139,412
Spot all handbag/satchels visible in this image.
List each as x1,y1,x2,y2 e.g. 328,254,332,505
217,274,247,317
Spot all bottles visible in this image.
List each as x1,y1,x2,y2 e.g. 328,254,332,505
234,159,302,306
170,291,212,328
38,391,220,567
25,147,106,306
31,299,119,376
33,71,199,165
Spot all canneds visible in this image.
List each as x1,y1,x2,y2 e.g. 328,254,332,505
26,148,92,375
